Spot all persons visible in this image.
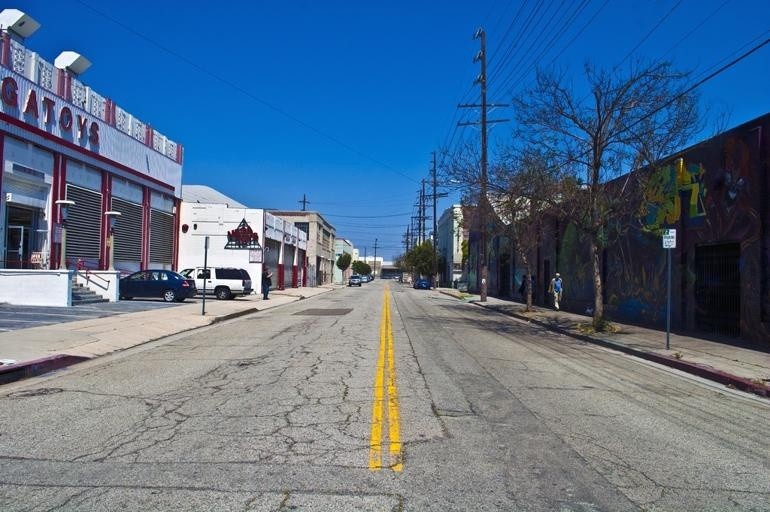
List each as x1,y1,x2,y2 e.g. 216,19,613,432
548,273,564,311
519,275,536,301
262,266,273,300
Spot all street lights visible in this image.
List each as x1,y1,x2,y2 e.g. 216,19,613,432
54,200,75,268
201,236,211,315
449,178,487,302
105,211,121,270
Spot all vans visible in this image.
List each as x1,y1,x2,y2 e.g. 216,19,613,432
177,267,254,300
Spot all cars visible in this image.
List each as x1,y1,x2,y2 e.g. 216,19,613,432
393,275,400,279
119,269,198,302
413,278,430,289
361,276,375,283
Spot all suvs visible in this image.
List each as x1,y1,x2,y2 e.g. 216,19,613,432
348,275,361,286
